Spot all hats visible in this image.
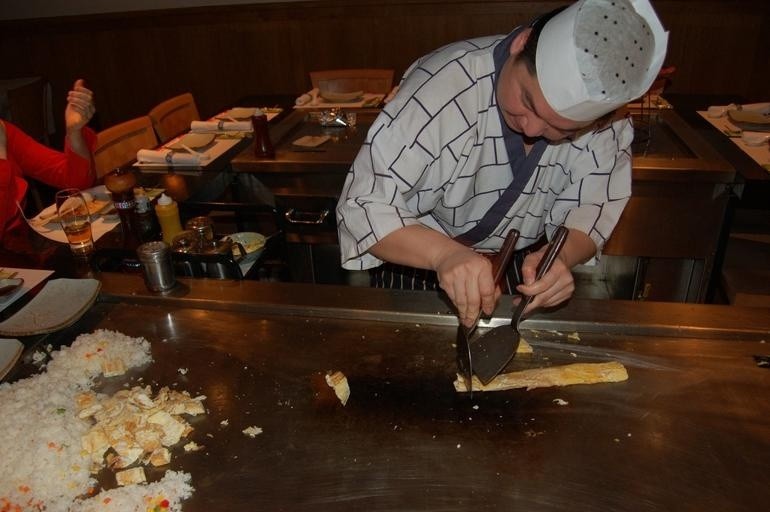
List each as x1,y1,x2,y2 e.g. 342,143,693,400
535,0,670,122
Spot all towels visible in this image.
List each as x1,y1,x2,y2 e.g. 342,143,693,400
294,87,318,105
135,147,197,165
707,102,770,117
190,118,253,132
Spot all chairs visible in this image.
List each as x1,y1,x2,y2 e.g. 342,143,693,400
7,69,51,211
88,116,160,186
308,67,396,104
148,92,201,144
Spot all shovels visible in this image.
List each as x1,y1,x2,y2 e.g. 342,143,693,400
469,226,569,385
456,228,520,400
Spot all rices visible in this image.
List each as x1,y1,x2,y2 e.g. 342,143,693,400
0,327,195,512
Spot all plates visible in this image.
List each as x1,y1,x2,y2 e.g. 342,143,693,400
1,278,106,387
165,103,259,152
706,100,769,156
318,90,368,102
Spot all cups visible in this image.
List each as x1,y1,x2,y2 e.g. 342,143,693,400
55,187,96,261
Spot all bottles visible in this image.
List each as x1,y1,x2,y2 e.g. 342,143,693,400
251,109,275,159
103,166,247,292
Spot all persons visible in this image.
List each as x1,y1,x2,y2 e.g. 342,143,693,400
335,0,670,328
0,78,96,274
